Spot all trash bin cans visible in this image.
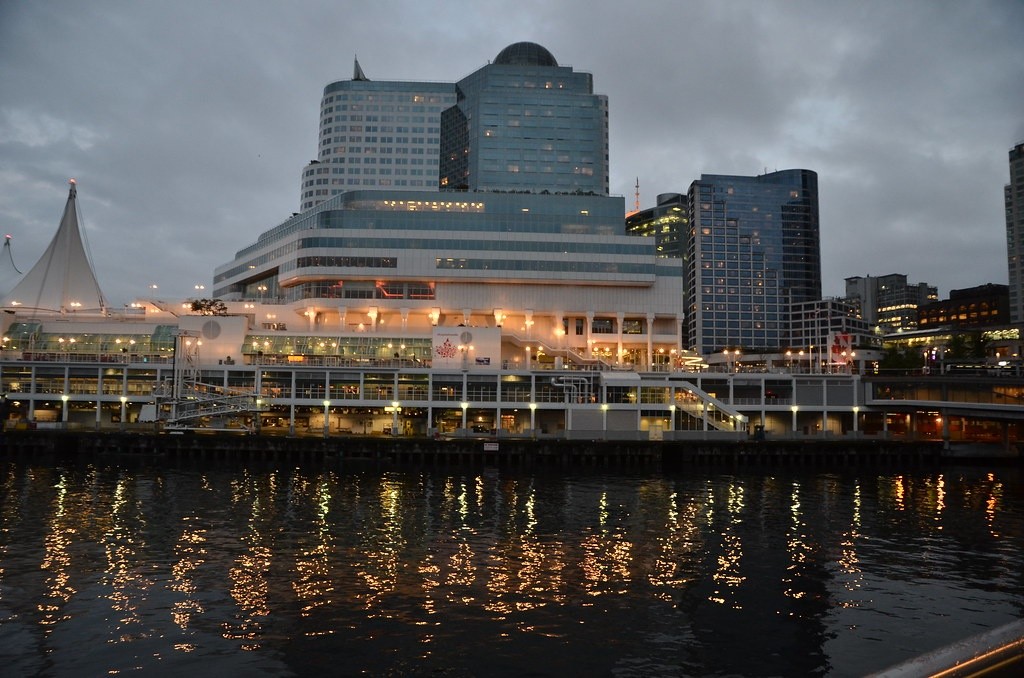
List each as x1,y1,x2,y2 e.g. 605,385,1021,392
753,425,765,440
